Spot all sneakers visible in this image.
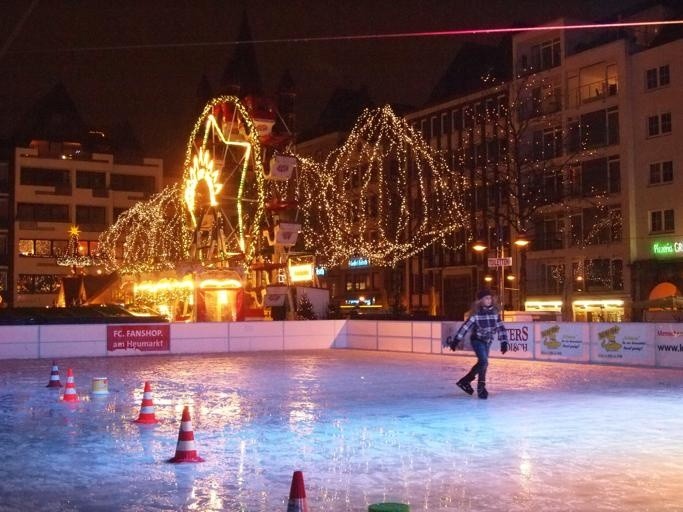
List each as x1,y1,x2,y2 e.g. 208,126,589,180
460,372,475,393
476,382,488,397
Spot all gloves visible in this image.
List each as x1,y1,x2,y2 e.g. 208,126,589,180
500,341,508,354
450,335,460,351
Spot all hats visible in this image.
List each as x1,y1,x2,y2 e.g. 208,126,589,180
477,290,493,299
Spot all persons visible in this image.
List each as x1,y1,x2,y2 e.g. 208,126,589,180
449,289,510,399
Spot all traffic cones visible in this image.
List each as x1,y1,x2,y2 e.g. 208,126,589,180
46,361,63,387
169,406,203,462
131,383,160,424
286,471,309,511
61,369,79,401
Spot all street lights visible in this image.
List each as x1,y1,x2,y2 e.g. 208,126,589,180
472,236,530,321
